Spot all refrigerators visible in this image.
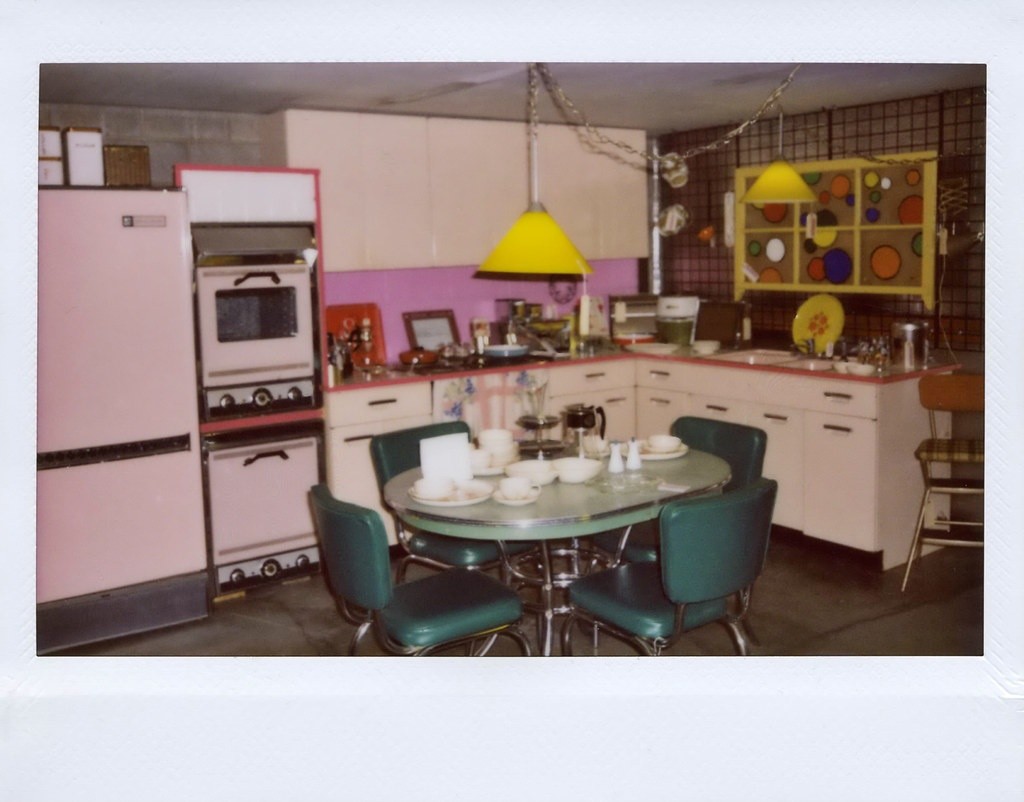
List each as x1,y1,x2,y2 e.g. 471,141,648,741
39,186,208,654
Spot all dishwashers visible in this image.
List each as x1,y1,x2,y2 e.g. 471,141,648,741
209,436,324,586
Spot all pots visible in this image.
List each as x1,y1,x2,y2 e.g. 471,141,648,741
400,345,438,365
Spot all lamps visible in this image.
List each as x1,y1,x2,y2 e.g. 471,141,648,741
738,104,819,204
475,61,593,275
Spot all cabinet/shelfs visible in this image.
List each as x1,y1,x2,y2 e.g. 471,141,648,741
635,356,684,439
324,381,432,547
548,358,635,439
803,374,955,572
431,365,548,441
690,392,803,533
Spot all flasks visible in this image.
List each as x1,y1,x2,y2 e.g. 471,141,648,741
890,320,927,370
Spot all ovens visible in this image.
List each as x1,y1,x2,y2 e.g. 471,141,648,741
201,264,312,408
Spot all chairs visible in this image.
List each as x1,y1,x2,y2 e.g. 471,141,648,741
560,477,778,656
579,415,767,566
899,371,984,594
310,483,533,657
374,419,541,585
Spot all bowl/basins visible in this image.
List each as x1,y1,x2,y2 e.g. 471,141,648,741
506,456,602,486
833,359,877,377
413,478,451,499
471,427,516,469
644,435,681,454
691,341,719,354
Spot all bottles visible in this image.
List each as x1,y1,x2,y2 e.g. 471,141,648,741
356,313,380,372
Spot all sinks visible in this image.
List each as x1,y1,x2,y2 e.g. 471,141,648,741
703,347,886,377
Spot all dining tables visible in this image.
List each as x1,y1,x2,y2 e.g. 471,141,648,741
384,438,735,657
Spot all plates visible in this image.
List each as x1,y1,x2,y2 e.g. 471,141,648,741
402,467,540,508
793,296,846,357
624,341,679,356
614,436,687,460
485,342,528,358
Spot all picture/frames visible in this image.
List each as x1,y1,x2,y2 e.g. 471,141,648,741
403,308,461,353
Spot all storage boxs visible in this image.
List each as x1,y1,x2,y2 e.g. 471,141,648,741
39,126,64,159
103,145,152,187
38,158,64,185
61,126,105,186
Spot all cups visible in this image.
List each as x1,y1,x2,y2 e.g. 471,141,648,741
499,476,542,500
657,295,700,348
563,403,608,459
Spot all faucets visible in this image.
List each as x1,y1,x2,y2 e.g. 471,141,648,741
786,337,815,356
